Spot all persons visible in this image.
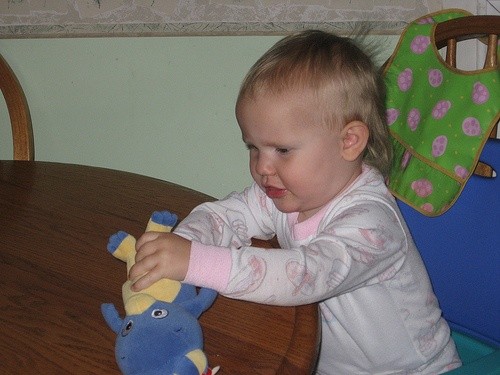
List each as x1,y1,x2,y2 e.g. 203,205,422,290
126,28,463,375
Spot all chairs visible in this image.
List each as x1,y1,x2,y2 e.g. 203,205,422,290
377,14,500,375
0,53,34,161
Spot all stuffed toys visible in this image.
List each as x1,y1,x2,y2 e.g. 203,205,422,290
99,211,221,375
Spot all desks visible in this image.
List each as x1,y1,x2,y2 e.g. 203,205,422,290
0,159,321,375
